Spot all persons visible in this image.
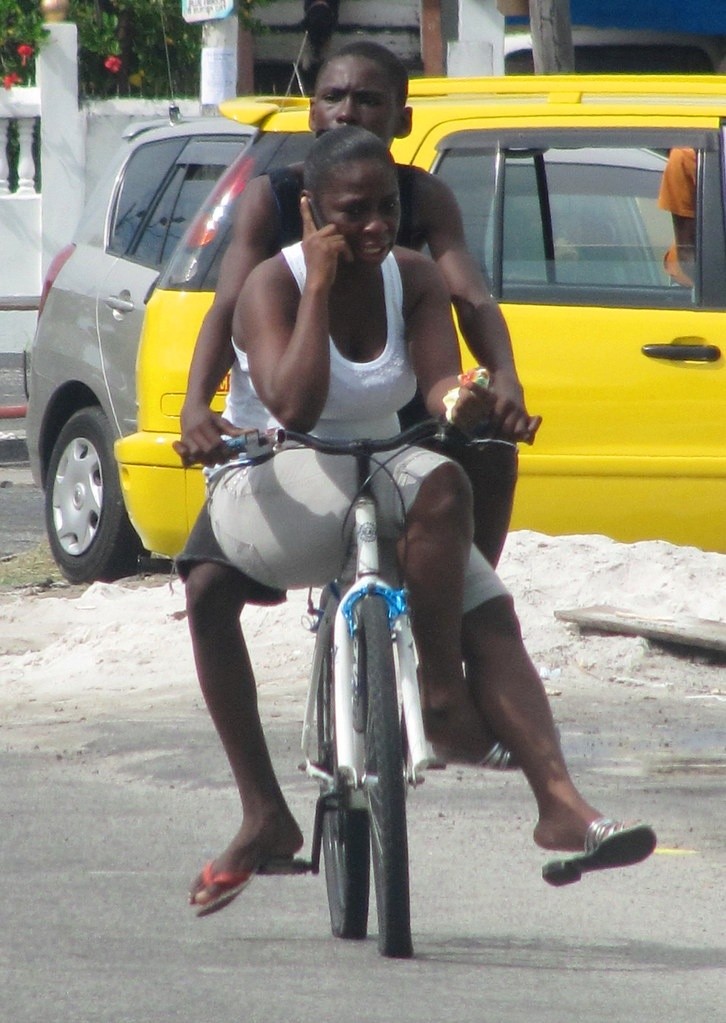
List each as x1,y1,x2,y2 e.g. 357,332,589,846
175,39,544,918
206,127,655,885
654,147,698,286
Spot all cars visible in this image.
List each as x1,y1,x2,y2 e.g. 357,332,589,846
116,76,725,557
25,114,700,586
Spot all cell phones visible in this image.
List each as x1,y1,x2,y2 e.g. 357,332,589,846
308,199,348,272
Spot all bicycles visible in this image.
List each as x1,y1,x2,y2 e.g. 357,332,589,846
179,410,541,958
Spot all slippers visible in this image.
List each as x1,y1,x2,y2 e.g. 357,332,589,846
432,740,522,772
194,855,294,917
542,816,657,887
